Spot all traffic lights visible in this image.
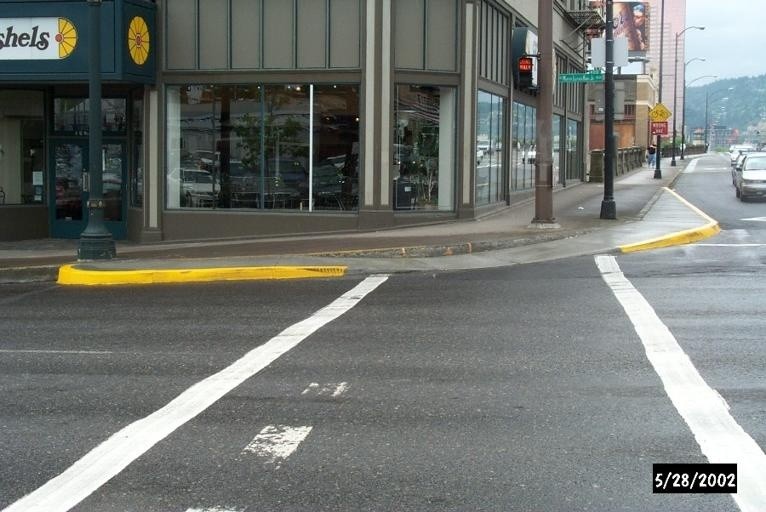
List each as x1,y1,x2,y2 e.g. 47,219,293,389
519,58,533,88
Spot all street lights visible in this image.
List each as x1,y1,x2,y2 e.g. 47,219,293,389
680,57,706,160
671,25,706,166
685,75,719,88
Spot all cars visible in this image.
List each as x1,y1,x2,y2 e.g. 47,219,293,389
730,144,766,202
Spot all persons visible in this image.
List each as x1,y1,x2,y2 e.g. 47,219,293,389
645,140,656,169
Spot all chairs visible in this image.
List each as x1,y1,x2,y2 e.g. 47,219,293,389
228,159,360,210
